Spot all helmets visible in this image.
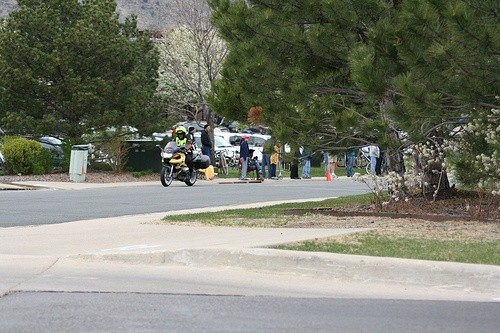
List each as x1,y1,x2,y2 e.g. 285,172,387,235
176,125,186,134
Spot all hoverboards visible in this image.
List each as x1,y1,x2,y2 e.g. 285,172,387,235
271,161,283,180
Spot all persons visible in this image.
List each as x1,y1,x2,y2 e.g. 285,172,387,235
162,127,195,149
171,126,193,178
201,125,213,159
240,133,385,182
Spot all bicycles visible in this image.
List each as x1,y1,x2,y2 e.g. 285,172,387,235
214,149,228,176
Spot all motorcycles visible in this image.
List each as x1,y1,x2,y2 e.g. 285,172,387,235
155,144,211,187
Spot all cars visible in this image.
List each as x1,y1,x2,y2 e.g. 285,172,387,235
194,121,271,164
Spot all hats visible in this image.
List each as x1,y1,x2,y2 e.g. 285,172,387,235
242,135,249,140
189,127,194,130
204,125,208,129
249,149,255,154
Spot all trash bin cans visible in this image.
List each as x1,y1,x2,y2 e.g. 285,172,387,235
68,145,88,183
